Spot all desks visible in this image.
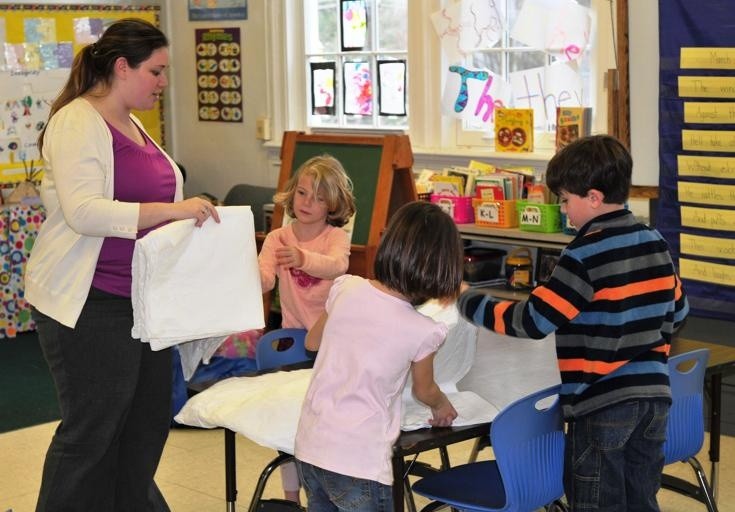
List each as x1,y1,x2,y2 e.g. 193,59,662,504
172,319,735,511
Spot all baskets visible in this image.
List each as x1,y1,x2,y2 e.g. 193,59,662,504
471,197,518,228
517,201,564,234
430,194,475,224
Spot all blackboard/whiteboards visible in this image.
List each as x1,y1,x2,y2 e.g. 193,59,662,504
272,132,395,250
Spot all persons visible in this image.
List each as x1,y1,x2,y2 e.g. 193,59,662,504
22,15,224,511
291,197,466,512
255,153,356,506
437,133,691,511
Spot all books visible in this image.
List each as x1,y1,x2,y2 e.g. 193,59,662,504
553,106,592,153
494,108,534,155
414,159,559,205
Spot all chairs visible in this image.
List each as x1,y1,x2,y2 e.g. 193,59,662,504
213,181,283,322
661,347,719,511
256,327,309,369
410,384,568,511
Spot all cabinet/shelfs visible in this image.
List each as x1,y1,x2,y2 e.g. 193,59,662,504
452,221,578,302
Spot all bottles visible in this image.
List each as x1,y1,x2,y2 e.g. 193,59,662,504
505,248,533,290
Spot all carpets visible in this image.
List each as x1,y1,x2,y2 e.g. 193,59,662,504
0,328,65,435
